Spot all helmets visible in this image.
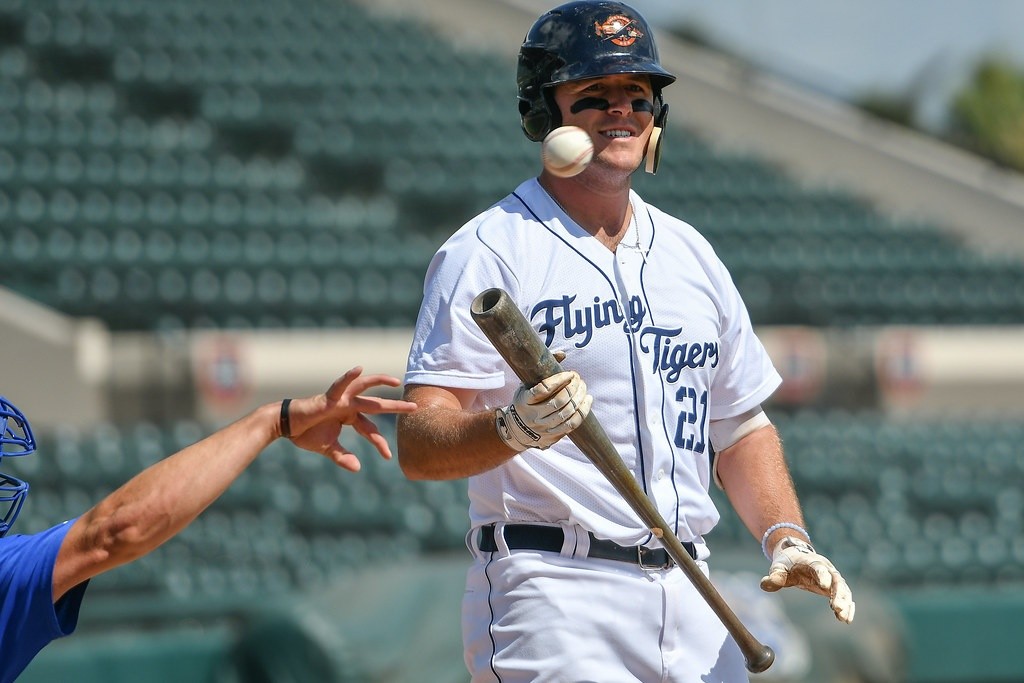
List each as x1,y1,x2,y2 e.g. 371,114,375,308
2,397,37,537
516,0,677,144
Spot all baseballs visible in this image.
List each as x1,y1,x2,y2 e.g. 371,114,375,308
540,124,598,179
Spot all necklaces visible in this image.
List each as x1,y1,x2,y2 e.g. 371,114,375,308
536,176,650,264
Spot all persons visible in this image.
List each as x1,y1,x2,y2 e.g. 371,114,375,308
0,364,417,683
397,0,855,683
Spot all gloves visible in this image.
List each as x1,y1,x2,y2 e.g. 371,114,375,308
758,538,857,625
496,349,596,453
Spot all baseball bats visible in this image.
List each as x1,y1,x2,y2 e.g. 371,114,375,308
469,286,778,674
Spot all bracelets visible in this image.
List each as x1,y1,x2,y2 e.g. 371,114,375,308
761,523,811,562
279,399,292,438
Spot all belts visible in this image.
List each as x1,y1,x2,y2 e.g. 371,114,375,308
470,524,696,570
